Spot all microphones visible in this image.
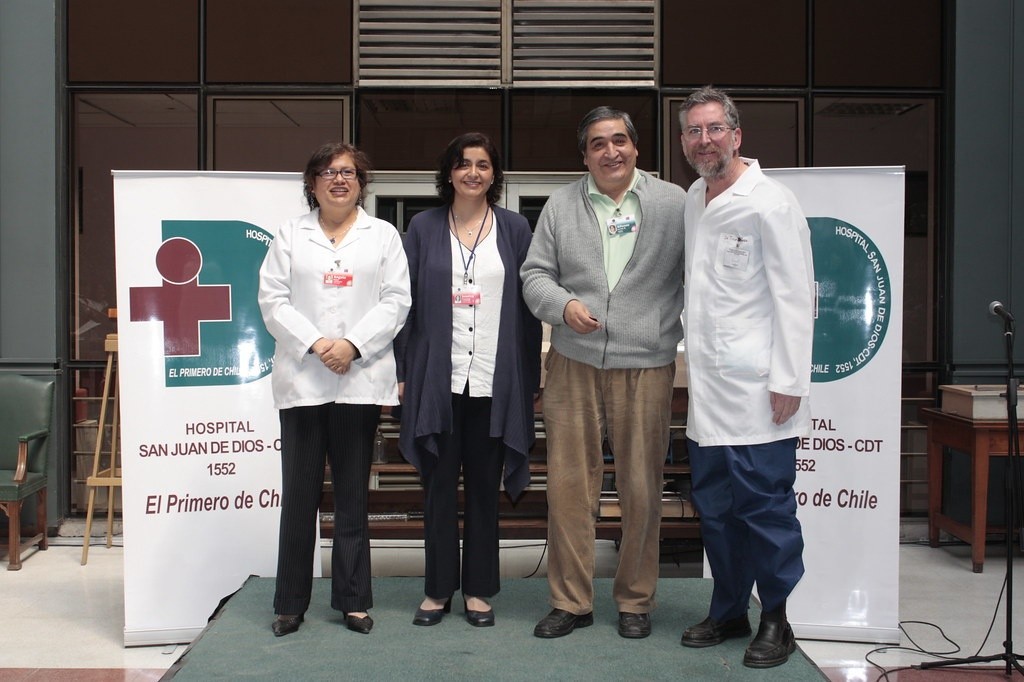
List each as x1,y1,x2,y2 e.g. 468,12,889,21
989,301,1015,322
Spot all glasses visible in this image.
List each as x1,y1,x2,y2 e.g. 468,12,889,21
681,120,737,142
315,168,358,181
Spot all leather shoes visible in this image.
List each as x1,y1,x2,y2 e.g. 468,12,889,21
617,610,652,639
342,610,374,633
744,617,797,667
681,611,753,647
271,612,305,637
534,607,596,637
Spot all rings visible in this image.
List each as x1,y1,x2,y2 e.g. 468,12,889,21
334,365,338,369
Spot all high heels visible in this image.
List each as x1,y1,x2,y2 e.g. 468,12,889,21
461,590,495,627
412,592,455,625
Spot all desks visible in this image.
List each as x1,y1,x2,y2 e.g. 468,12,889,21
922,408,1024,572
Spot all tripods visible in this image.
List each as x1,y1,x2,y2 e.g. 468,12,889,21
921,320,1024,676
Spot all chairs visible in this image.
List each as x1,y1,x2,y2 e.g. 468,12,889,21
0,373,55,571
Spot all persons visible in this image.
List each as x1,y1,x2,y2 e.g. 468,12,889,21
393,132,543,626
257,143,412,636
326,275,332,283
610,225,616,234
455,295,461,303
520,106,687,638
677,88,815,668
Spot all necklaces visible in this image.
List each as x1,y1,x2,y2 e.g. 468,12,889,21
454,213,485,236
320,217,352,244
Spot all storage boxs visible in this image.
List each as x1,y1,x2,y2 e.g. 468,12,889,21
939,385,1024,420
